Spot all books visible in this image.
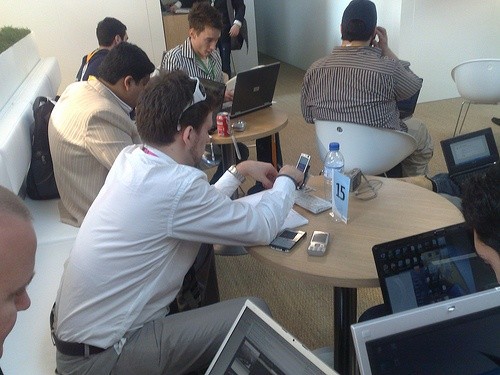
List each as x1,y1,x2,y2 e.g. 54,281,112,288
232,191,308,236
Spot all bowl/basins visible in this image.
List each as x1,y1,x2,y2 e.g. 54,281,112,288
232,120,248,132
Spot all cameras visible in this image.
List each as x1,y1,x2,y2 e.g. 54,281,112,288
345,168,362,193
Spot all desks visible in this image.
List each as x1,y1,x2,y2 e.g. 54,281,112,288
203,99,289,256
243,174,467,375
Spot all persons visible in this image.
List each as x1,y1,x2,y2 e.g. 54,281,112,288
48,41,220,316
74,16,130,82
202,1,246,82
158,2,251,188
50,70,306,375
0,185,37,375
356,159,500,322
299,0,435,177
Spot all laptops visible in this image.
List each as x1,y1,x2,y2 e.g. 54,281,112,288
395,78,423,121
200,222,500,374
440,126,500,189
191,62,281,134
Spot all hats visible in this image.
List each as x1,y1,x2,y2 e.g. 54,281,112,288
341,0,377,36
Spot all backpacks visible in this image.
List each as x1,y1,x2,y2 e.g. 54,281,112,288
28,96,60,201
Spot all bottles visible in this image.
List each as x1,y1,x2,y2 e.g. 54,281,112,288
323,142,345,203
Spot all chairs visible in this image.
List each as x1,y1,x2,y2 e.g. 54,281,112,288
450,58,500,139
311,119,420,180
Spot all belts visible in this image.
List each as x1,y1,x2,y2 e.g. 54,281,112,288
49,302,106,356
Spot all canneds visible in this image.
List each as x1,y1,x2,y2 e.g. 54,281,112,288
216,112,232,137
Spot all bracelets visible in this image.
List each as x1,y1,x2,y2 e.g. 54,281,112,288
276,174,297,186
227,163,246,184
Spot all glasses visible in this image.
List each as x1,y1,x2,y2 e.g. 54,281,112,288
177,76,206,133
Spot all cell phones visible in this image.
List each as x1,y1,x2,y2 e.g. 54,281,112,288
295,152,311,174
269,229,330,257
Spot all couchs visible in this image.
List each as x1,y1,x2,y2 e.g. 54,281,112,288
0,54,81,375
